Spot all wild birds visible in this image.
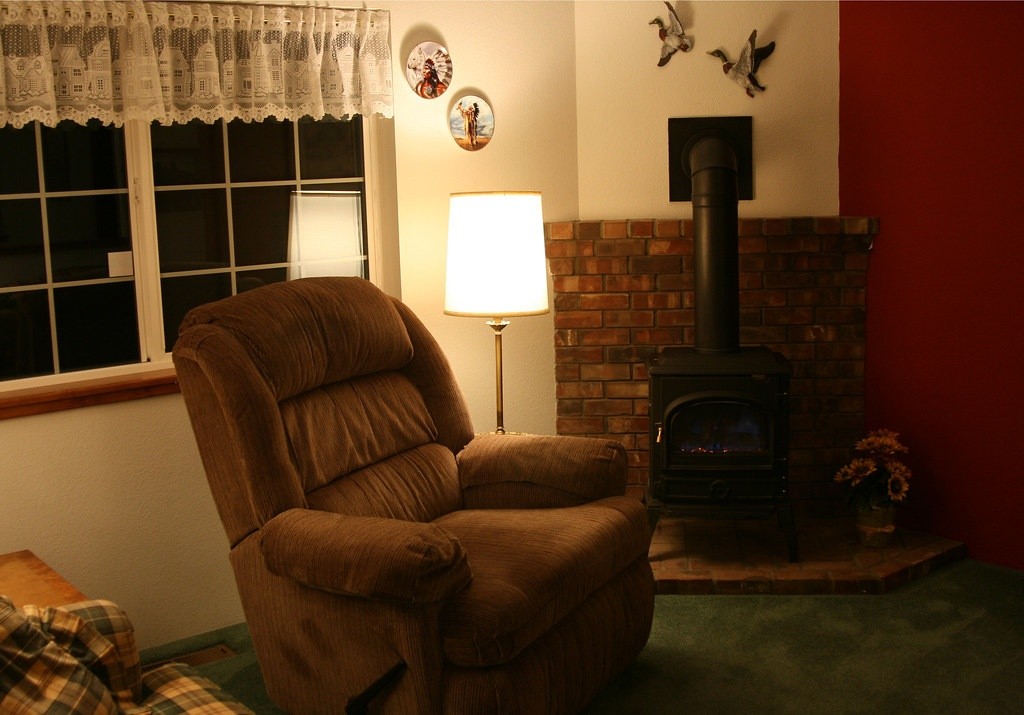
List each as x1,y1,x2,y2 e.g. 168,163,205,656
706,28,776,99
648,1,693,68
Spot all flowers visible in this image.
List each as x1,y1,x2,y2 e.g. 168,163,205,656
835,428,913,510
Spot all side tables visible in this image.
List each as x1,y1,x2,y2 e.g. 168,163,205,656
0,549,90,609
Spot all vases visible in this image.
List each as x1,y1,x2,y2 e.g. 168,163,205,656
855,503,898,549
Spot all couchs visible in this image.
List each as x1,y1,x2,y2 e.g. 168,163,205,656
0,593,255,715
170,275,656,715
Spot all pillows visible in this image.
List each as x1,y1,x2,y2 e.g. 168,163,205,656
0,595,152,715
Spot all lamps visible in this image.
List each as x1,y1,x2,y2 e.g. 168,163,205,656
285,191,365,281
444,191,551,435
